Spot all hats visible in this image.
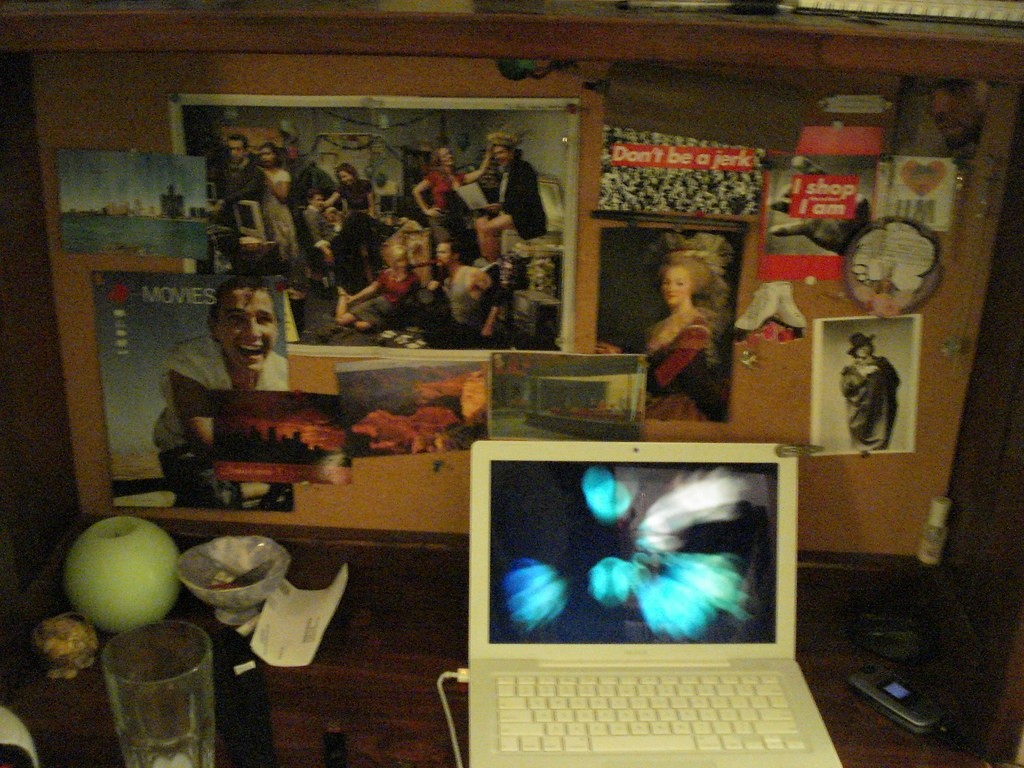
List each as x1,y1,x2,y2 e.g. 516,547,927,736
845,332,876,355
485,133,517,147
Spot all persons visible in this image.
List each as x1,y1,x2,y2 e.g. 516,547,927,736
840,332,901,453
219,129,733,422
148,278,295,511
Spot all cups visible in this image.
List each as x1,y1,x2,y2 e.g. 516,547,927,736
104,621,215,768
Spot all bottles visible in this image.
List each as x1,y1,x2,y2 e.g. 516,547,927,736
915,497,951,565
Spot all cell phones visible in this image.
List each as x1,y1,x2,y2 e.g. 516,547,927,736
845,660,956,734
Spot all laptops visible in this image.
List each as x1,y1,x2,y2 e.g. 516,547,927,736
468,440,842,768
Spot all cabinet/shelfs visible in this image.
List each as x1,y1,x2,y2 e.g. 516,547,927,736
0,0,1024,768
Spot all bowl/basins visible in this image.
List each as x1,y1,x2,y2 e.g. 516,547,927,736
179,535,290,625
